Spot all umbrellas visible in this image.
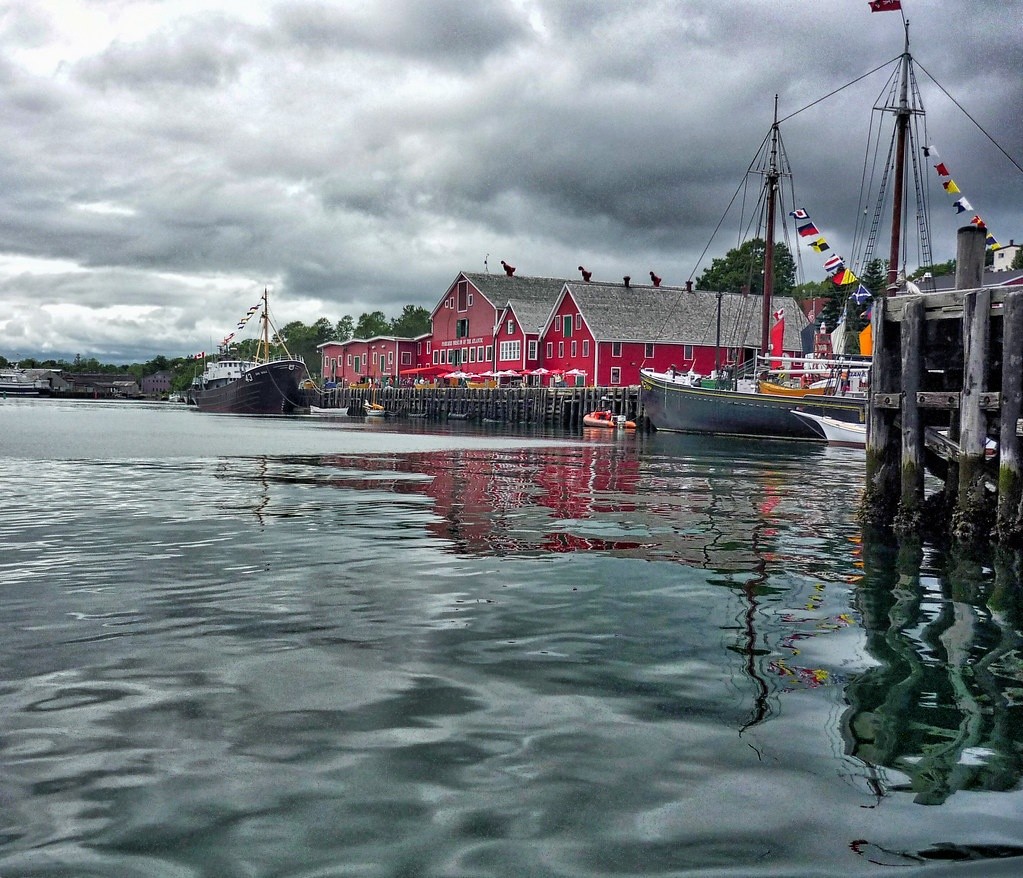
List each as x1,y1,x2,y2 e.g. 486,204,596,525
436,368,565,387
562,368,588,385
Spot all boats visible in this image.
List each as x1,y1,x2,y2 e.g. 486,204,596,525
310,404,350,417
168,393,181,403
582,400,637,430
790,405,869,449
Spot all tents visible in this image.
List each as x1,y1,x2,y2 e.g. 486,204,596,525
400,367,450,384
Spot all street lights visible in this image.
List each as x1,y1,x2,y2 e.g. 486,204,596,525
194,364,202,389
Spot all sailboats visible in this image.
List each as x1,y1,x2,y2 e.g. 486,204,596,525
635,92,872,443
363,363,386,417
749,0,1023,398
187,283,307,418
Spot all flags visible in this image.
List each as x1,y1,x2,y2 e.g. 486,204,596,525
237,307,258,329
798,222,820,237
772,308,784,321
933,163,949,176
833,268,857,285
942,179,960,194
848,284,871,305
921,145,937,157
953,197,973,214
860,301,876,319
868,0,901,13
195,352,204,359
807,237,830,253
985,233,1000,250
224,333,235,343
822,253,845,272
970,216,985,227
789,208,810,219
